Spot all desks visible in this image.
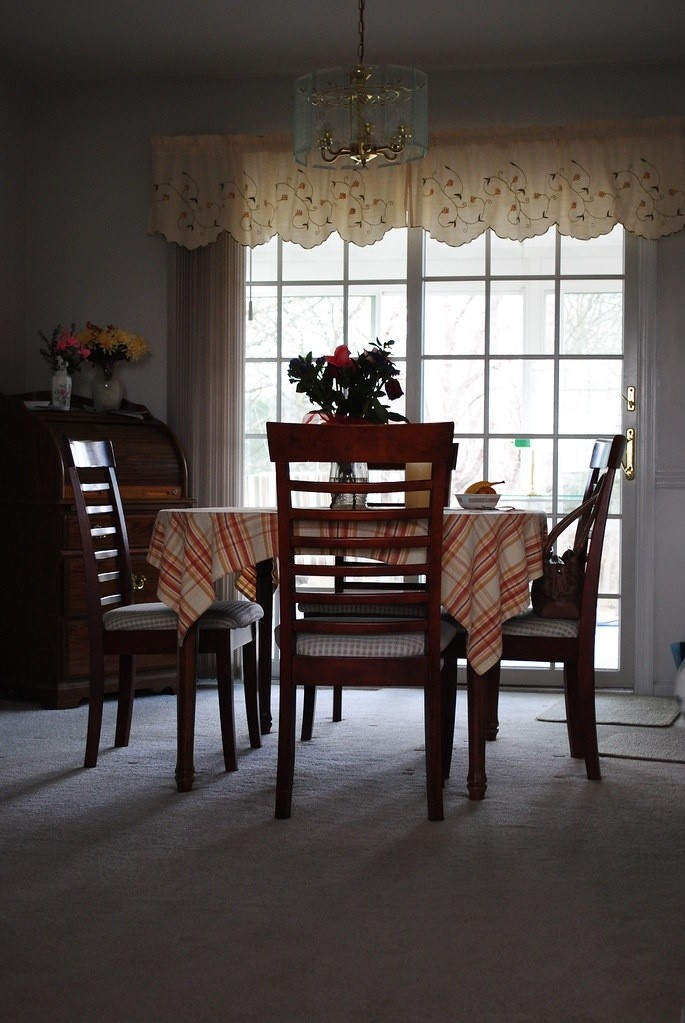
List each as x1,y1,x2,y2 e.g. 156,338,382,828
148,506,548,801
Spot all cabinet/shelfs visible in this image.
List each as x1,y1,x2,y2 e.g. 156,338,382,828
0,391,196,710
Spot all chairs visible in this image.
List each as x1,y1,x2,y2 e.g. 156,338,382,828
443,433,628,781
334,442,460,722
265,419,456,823
54,434,264,793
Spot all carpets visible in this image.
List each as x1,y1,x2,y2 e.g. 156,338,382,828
536,691,684,726
598,732,685,764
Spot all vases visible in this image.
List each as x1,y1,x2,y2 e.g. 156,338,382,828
329,462,368,510
52,370,72,411
91,370,123,412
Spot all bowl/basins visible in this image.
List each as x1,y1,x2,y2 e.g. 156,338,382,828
24,401,51,410
454,494,502,510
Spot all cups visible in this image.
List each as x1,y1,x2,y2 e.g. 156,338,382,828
406,463,432,508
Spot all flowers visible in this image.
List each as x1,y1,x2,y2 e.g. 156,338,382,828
38,321,91,375
288,336,409,509
77,321,149,380
